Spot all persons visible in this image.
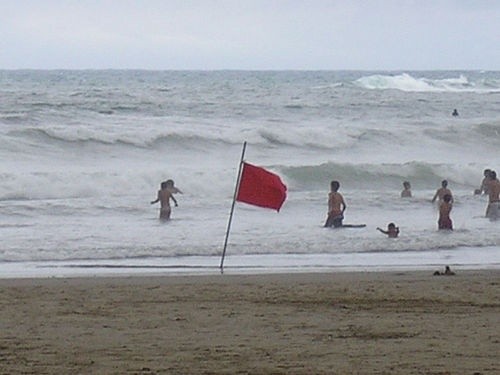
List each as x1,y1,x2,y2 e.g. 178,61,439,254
431,180,454,204
485,170,500,218
480,169,492,195
150,182,178,219
438,194,455,231
376,222,400,238
452,108,458,116
165,179,184,195
401,182,412,197
323,180,346,228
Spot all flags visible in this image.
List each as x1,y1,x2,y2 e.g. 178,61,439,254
236,163,287,213
